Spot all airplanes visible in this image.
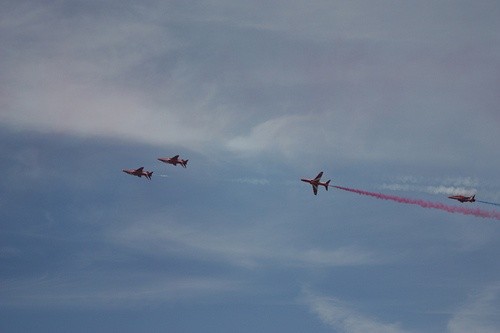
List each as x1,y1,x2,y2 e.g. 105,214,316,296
448,194,476,204
157,155,188,168
122,166,153,181
299,171,330,196
